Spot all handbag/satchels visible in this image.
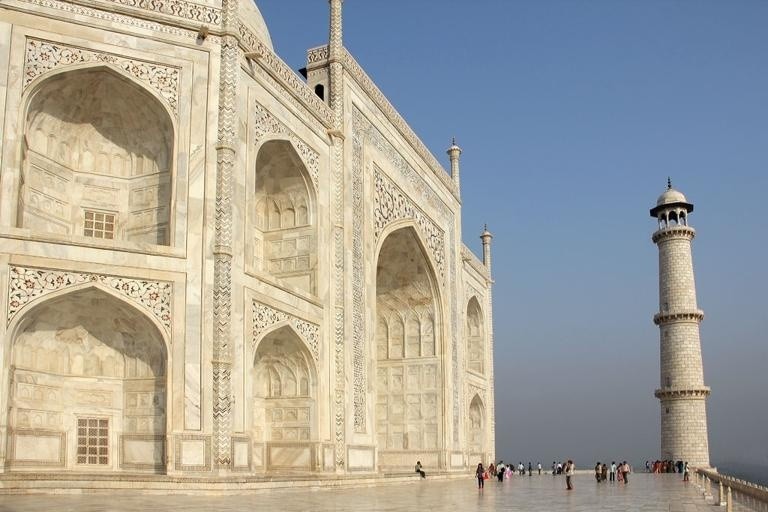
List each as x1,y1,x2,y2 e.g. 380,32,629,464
482,473,488,480
501,468,505,472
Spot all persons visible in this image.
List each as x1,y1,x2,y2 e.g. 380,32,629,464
528,462,532,476
646,459,691,481
551,459,575,490
537,461,541,474
488,460,526,482
475,463,487,489
595,461,631,484
415,460,426,478
